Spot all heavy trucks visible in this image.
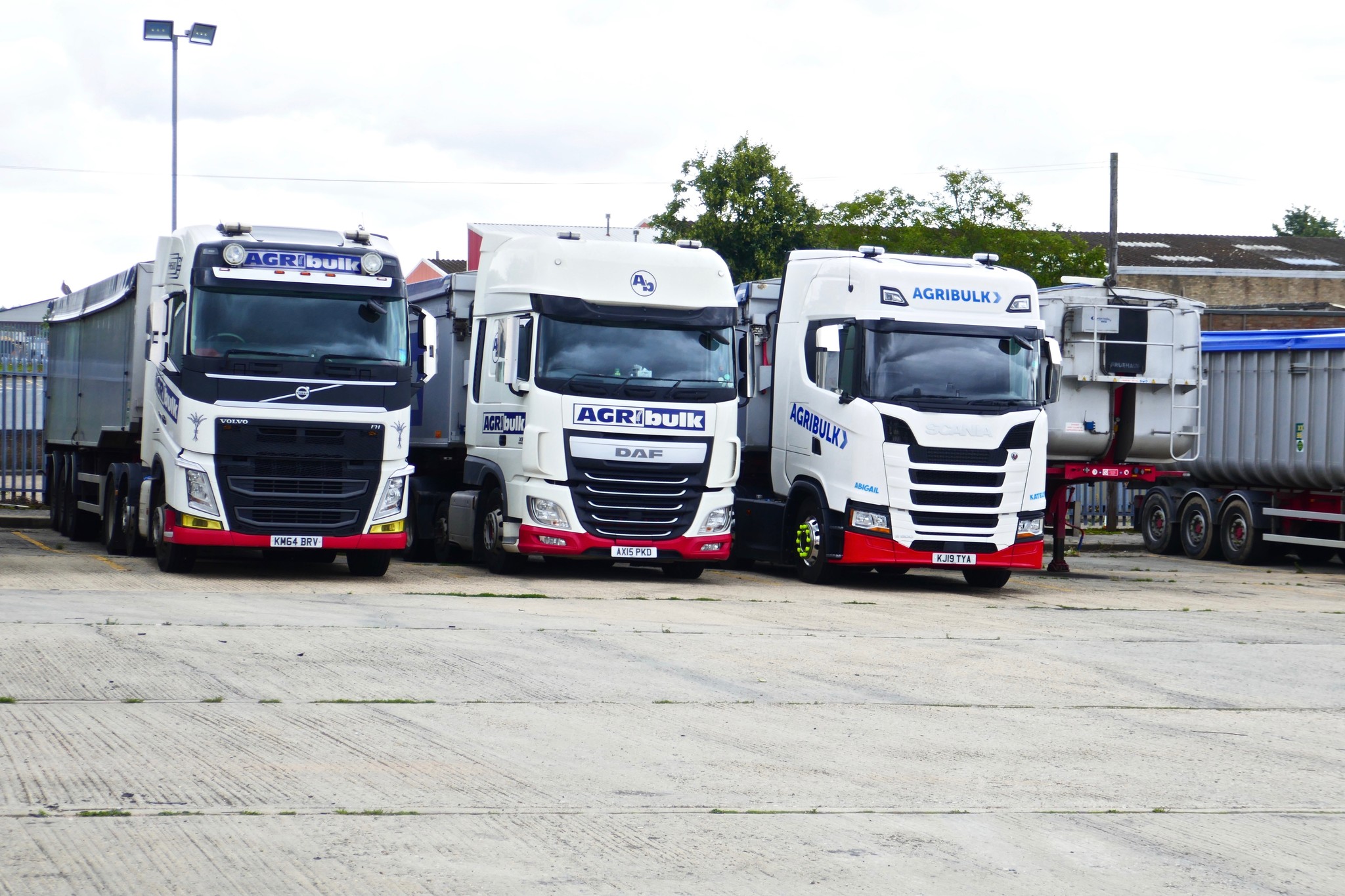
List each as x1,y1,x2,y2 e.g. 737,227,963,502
45,224,437,577
406,231,756,583
700,246,1064,590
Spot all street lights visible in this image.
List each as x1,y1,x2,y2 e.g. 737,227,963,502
142,19,218,229
